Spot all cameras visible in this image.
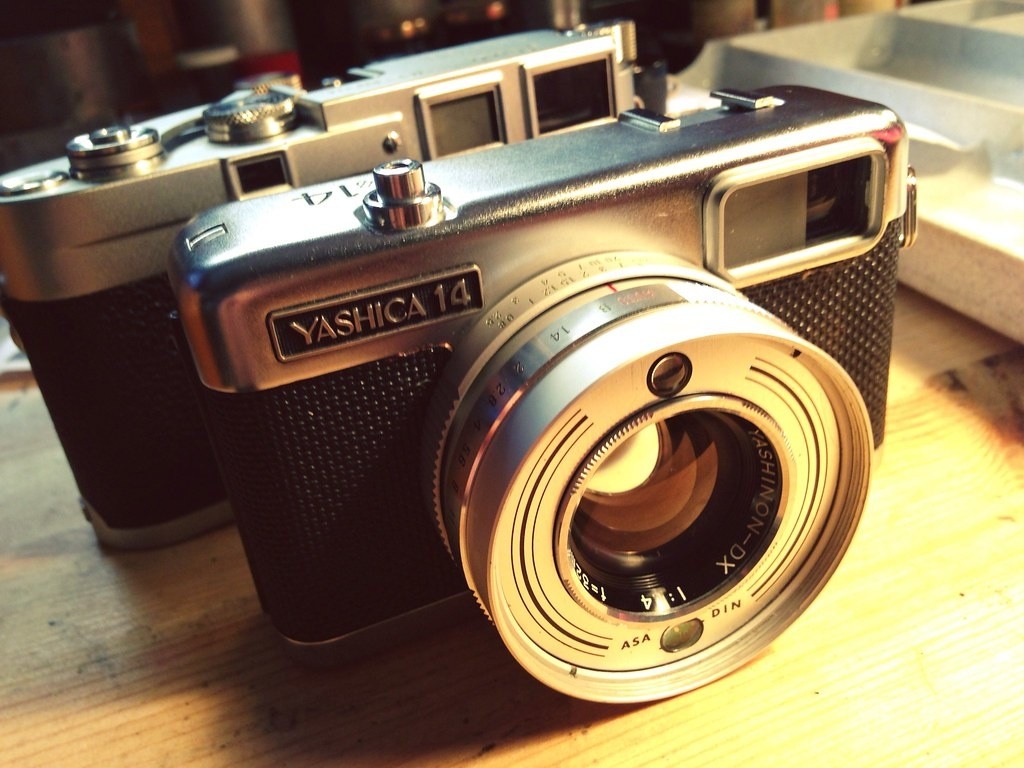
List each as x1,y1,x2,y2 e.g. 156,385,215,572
0,21,916,704
